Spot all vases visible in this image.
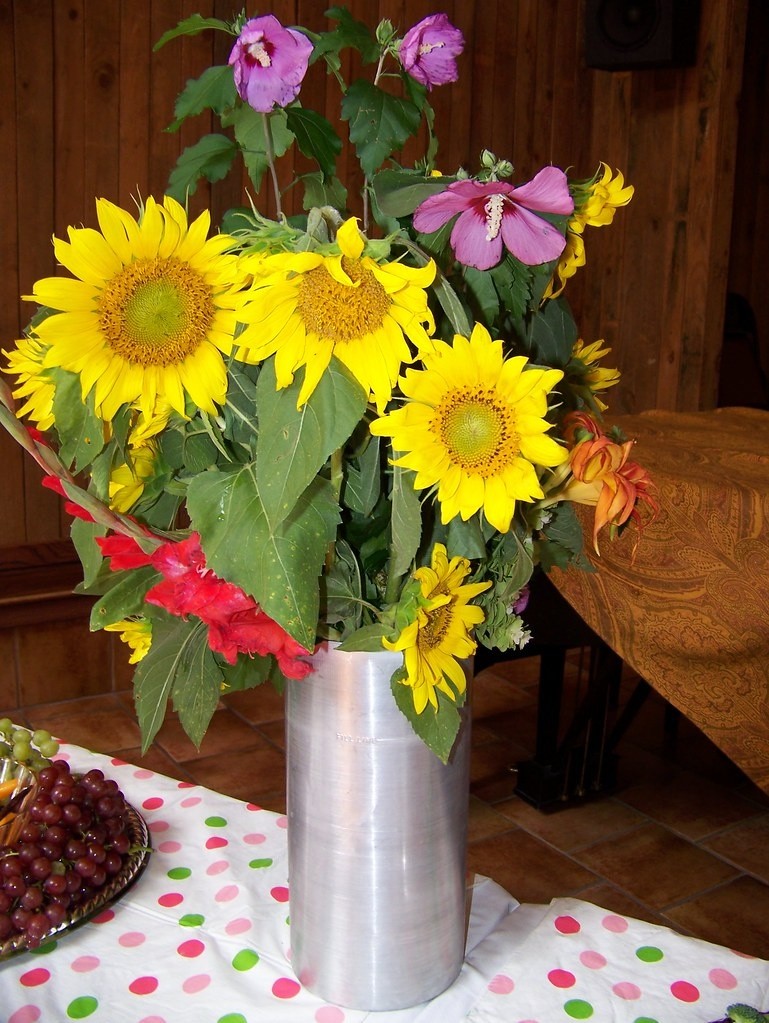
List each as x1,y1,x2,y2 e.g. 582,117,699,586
287,641,471,1011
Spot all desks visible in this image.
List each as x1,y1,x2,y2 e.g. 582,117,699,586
509,409,769,815
0,724,769,1023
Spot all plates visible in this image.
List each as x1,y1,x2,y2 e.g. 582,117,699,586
0,773,151,962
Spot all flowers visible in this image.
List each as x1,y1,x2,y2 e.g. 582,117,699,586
0,8,659,768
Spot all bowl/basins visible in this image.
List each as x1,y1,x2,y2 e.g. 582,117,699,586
0,758,36,849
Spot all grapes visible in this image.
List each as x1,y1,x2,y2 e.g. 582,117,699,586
0,717,133,951
